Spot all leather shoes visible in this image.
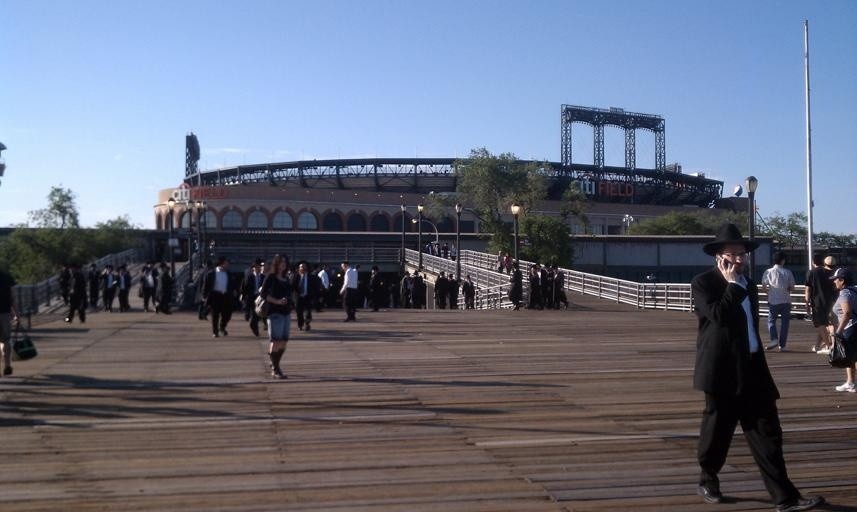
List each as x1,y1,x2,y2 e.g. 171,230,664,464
774,494,825,512
697,482,724,504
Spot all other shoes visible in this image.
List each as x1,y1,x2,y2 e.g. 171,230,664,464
220,327,228,336
298,326,303,331
512,303,568,311
213,333,219,338
344,316,356,322
63,301,208,323
306,323,311,330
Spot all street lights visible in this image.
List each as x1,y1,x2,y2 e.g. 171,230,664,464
417,202,424,272
454,200,461,284
411,219,438,250
622,214,632,234
196,200,202,268
510,202,520,270
744,175,756,284
202,200,207,265
188,198,192,283
401,202,405,263
167,196,175,278
0,143,7,186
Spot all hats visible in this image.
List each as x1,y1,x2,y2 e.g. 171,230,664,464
823,255,838,271
701,223,761,257
828,267,853,282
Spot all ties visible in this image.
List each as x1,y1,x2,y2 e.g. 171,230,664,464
258,275,260,290
299,274,305,295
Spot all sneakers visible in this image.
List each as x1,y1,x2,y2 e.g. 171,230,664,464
765,343,779,350
816,344,832,356
812,344,822,353
778,345,784,352
835,381,857,393
3,366,13,375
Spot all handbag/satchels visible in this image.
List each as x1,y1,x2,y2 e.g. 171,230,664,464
252,293,272,319
11,322,38,362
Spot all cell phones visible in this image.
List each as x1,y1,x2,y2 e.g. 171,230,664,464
716,257,729,267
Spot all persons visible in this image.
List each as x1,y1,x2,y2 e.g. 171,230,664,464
260,253,295,378
497,251,569,310
58,263,132,323
139,260,176,315
391,270,474,309
193,256,272,337
691,224,825,512
290,260,390,331
0,268,18,376
762,251,796,353
804,254,857,393
423,243,456,261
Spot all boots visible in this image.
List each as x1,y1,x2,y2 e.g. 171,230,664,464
269,348,286,368
268,351,287,379
